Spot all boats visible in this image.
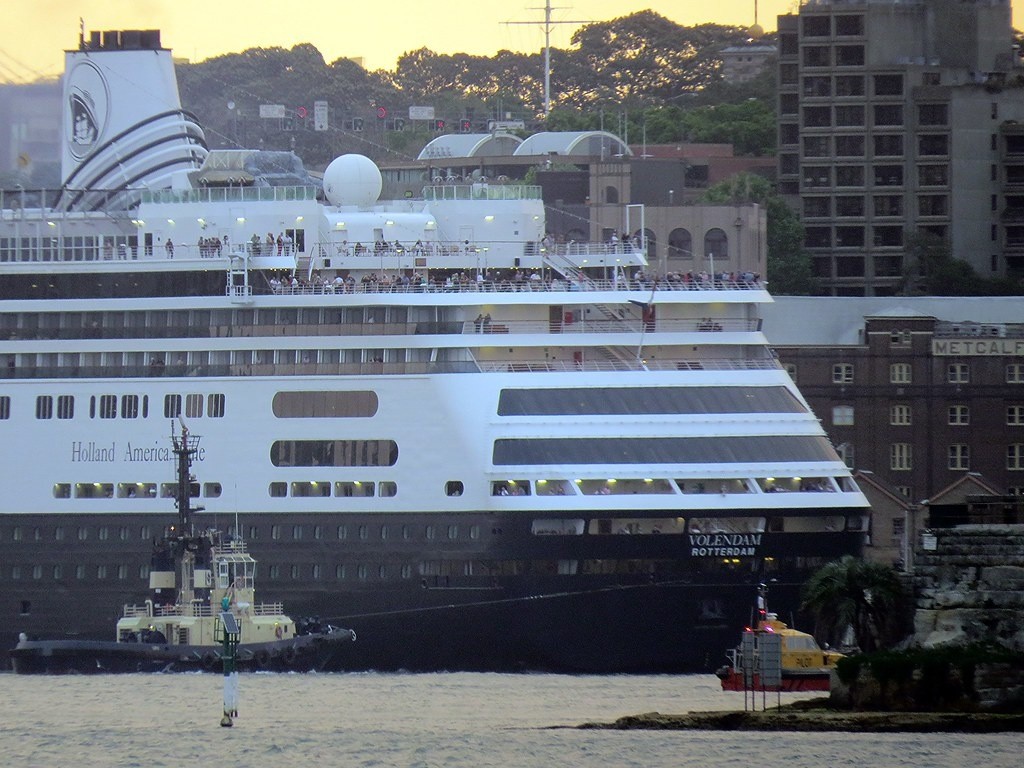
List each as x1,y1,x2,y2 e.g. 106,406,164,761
10,412,363,675
716,577,848,692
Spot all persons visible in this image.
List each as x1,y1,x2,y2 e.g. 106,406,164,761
369,356,384,374
451,482,611,497
302,356,310,375
102,238,137,260
699,317,720,331
6,357,17,378
148,630,166,643
19,630,41,642
198,232,292,259
533,233,637,256
271,271,762,295
104,487,196,498
295,614,321,637
474,313,492,333
462,172,510,197
148,356,184,377
165,238,175,259
89,318,102,339
719,480,835,494
336,232,477,258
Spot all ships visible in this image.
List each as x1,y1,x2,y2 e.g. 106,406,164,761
0,2,879,670
